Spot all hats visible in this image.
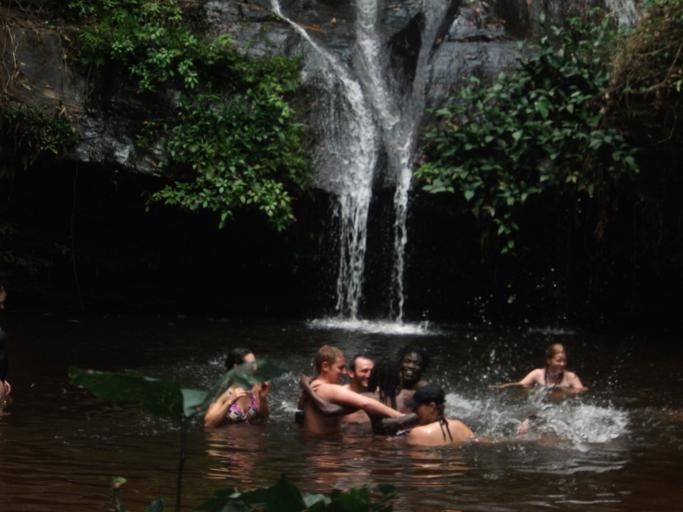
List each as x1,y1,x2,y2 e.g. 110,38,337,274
404,386,444,407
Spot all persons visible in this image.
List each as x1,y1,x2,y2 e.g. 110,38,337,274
297,341,587,447
205,347,271,428
